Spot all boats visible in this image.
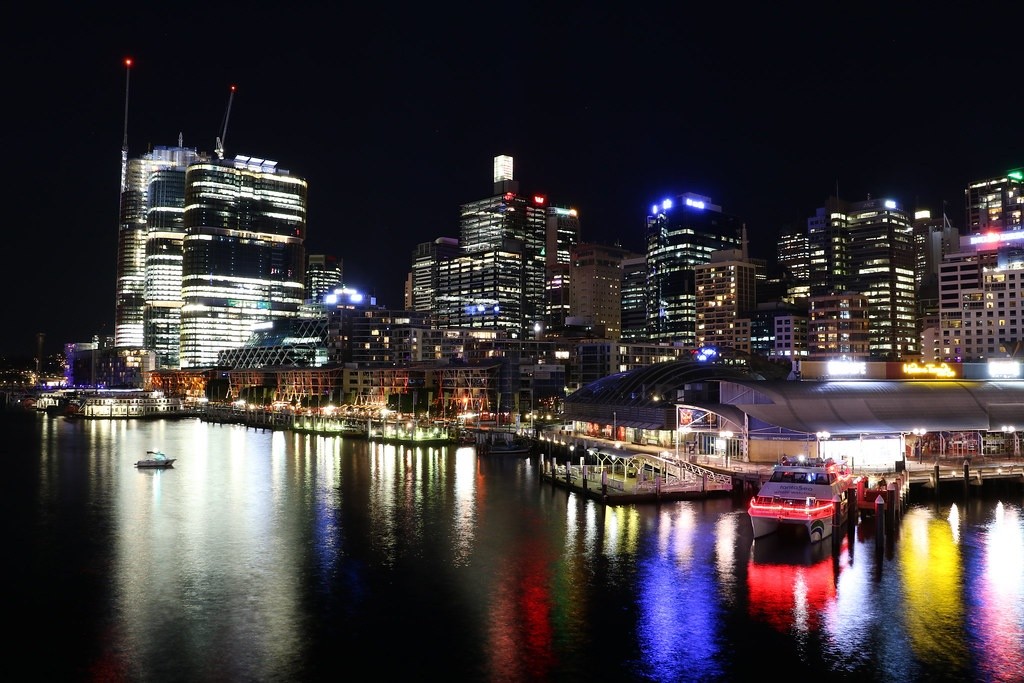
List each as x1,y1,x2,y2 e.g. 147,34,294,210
480,442,531,453
747,457,849,544
135,460,176,466
